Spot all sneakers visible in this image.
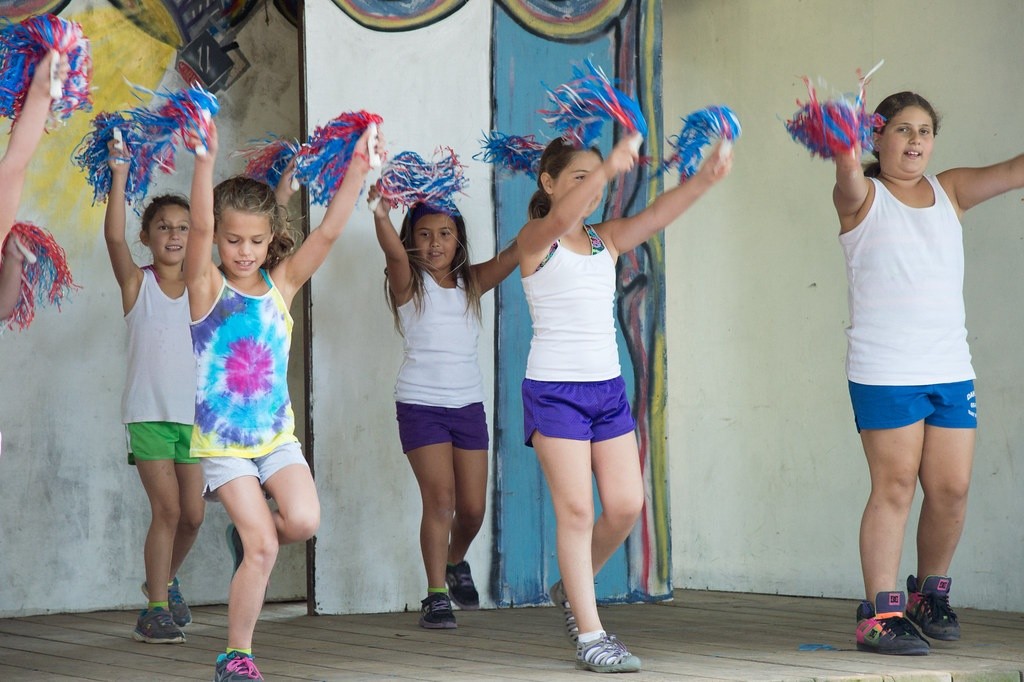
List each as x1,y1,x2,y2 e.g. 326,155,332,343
855,591,930,655
905,574,961,641
142,575,192,626
215,650,264,682
445,559,480,610
420,592,458,628
133,606,187,644
226,523,268,599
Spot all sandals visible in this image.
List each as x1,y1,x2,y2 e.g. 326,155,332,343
575,634,641,673
549,578,581,647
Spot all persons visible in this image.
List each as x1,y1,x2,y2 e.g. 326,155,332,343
1,47,70,243
183,117,386,681
519,129,735,672
1,230,30,319
833,92,1024,656
104,136,306,644
368,179,519,629
